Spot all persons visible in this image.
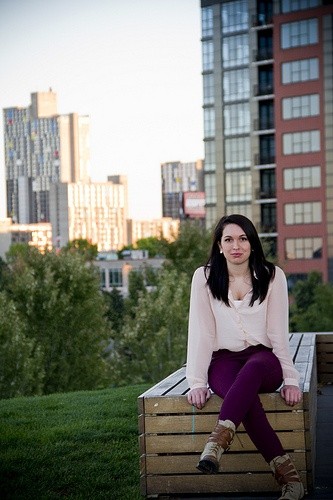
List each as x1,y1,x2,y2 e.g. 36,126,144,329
184,214,307,500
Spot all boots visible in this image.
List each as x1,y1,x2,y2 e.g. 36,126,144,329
195,420,236,474
269,453,305,500
